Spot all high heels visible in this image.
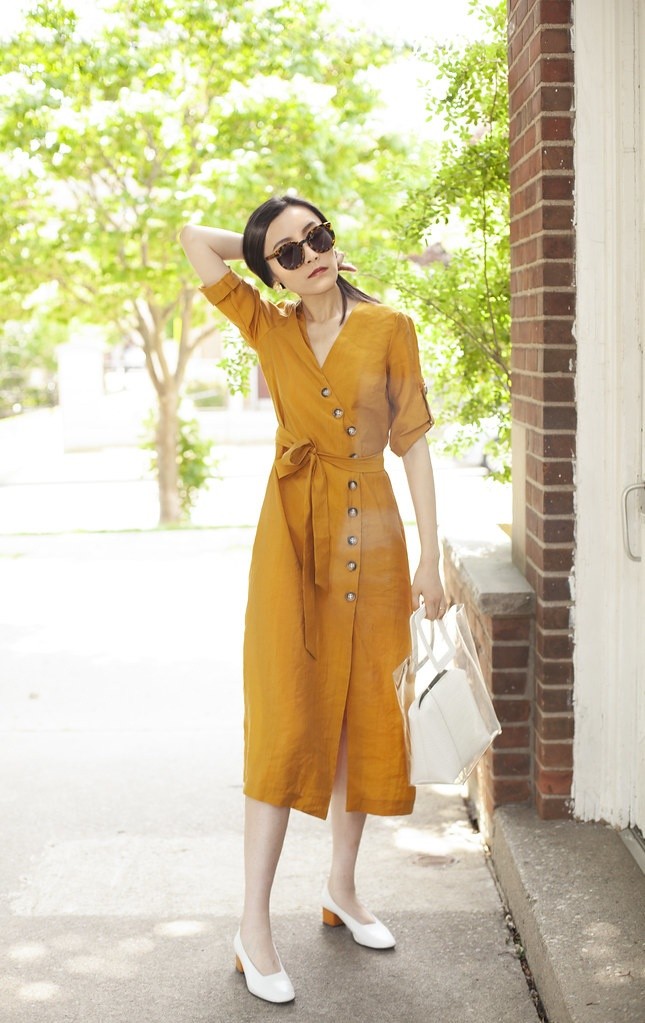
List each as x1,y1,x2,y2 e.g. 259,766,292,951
236,929,296,1003
322,886,394,949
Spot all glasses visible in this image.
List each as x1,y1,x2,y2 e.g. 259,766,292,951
265,220,335,271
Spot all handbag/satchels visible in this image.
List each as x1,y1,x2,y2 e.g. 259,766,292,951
391,596,502,787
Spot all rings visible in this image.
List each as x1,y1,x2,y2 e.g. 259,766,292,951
438,607,447,612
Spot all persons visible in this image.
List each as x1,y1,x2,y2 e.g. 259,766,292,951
182,196,447,1004
